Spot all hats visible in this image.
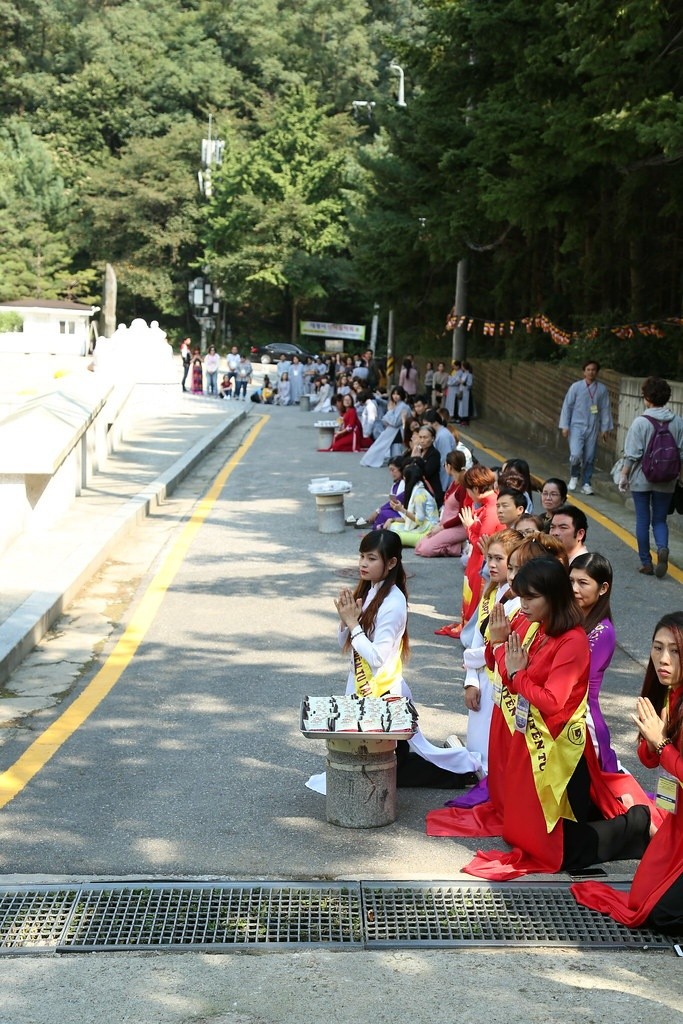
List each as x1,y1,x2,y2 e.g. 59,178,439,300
183,335,192,339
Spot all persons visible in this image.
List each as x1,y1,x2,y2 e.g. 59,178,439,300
317,386,683,943
618,378,683,578
180,338,475,427
559,361,609,496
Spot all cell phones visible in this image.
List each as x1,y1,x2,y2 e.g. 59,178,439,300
389,494,397,503
566,867,608,878
673,944,683,957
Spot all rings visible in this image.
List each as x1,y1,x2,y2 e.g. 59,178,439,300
489,621,493,624
514,648,517,652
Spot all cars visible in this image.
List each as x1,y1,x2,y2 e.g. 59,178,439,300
251,342,319,365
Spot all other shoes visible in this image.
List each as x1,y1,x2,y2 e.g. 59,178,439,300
183,388,188,392
235,396,239,401
638,564,654,576
450,417,468,425
656,549,668,578
242,397,245,400
228,395,230,399
224,396,227,400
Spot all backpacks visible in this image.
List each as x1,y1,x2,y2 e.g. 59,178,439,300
642,414,680,484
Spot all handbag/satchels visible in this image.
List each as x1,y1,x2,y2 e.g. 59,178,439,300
248,378,251,384
457,390,463,401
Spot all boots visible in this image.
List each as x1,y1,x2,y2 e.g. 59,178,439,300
589,805,650,862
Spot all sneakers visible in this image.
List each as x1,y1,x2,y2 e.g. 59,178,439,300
568,477,578,492
580,483,594,495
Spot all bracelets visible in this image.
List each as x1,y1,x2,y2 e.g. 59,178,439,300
349,630,365,644
655,737,673,754
490,639,505,647
510,670,519,683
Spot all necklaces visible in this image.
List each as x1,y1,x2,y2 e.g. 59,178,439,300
536,629,550,643
